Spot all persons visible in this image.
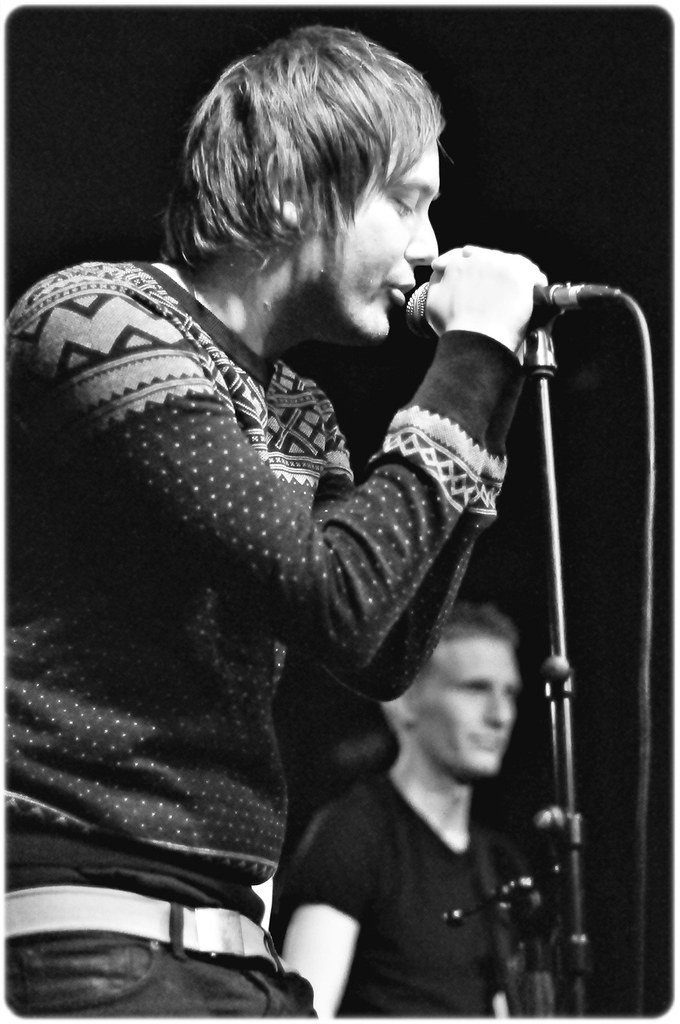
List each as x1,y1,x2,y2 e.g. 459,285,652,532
6,24,549,1020
275,597,523,1019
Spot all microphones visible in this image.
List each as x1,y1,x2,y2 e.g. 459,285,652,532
404,283,625,341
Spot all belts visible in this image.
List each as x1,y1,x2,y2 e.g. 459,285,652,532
3,884,289,974
